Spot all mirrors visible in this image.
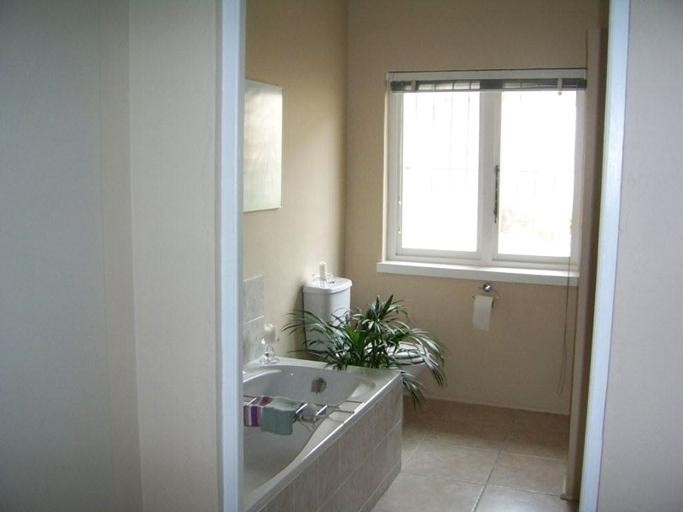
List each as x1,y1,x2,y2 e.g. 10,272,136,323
243,76,283,213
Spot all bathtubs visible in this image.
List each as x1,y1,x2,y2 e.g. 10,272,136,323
243,352,403,509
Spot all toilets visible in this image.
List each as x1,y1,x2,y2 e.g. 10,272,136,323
303,274,429,388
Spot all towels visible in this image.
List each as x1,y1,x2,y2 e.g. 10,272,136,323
261,396,306,435
243,396,273,426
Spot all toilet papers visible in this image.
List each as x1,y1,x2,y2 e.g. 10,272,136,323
472,295,494,331
